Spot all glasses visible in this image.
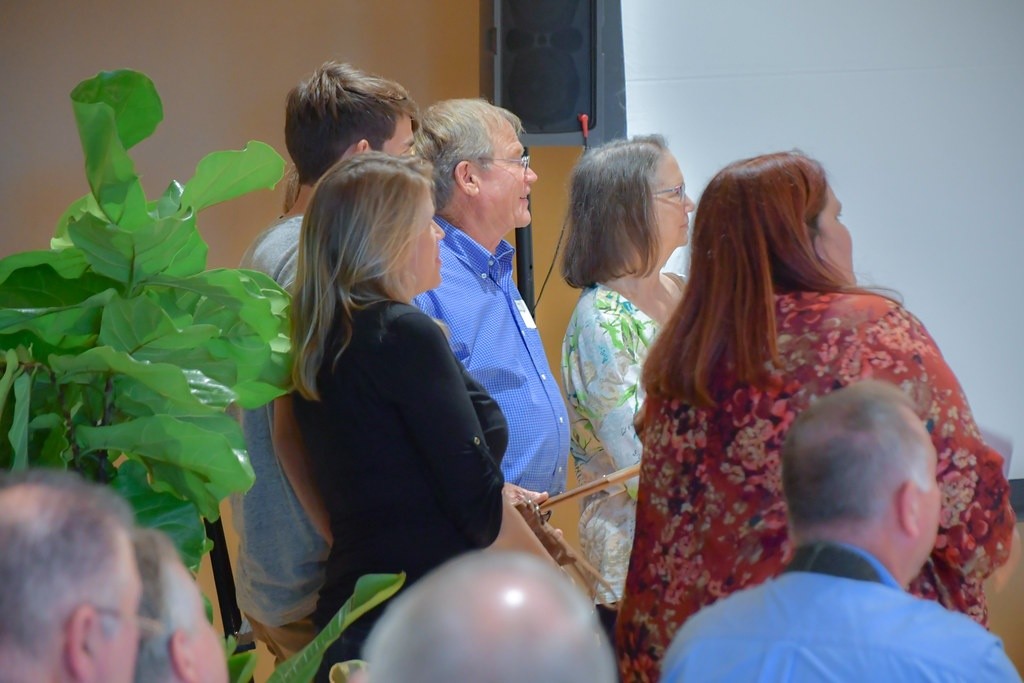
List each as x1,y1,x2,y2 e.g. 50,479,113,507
478,156,530,176
652,183,685,202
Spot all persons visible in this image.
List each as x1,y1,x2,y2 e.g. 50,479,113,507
0,63,1024,683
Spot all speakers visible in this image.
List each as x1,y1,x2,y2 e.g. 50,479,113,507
479,0,604,147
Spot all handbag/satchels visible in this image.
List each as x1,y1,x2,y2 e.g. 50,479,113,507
514,501,619,610
